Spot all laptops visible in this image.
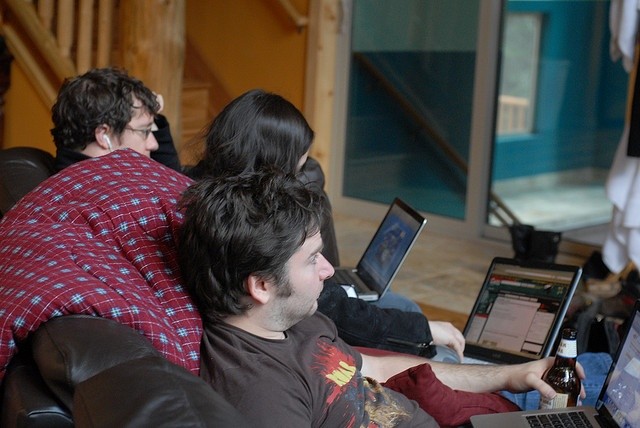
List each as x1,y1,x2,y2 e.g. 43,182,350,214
470,298,639,428
463,256,584,364
330,196,427,302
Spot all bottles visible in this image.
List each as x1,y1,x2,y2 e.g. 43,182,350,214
539,329,581,409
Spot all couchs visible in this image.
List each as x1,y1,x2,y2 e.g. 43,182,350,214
2,146,248,427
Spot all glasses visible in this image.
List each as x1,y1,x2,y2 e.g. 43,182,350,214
123,127,151,138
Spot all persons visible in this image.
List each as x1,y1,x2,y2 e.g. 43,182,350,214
46,66,340,269
188,87,469,363
173,165,612,428
